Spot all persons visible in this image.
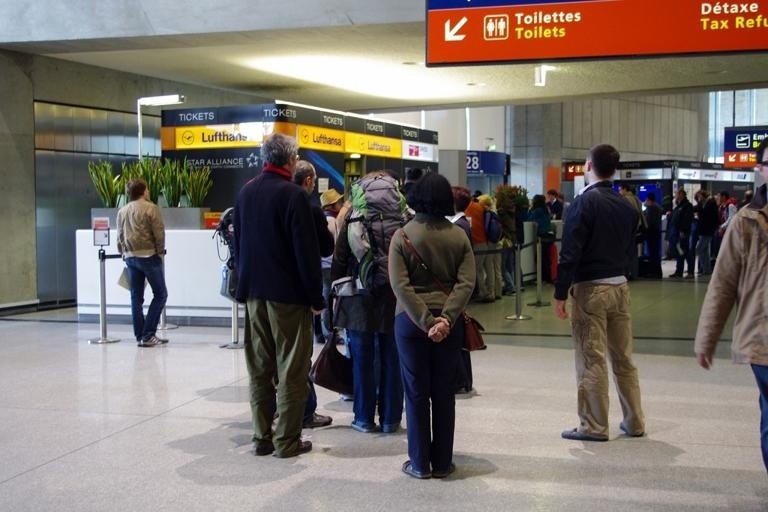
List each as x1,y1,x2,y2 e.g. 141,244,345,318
617,183,752,280
114,179,169,348
554,142,645,442
693,136,768,473
230,133,571,483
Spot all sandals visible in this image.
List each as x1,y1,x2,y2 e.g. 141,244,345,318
431,462,456,479
402,460,432,479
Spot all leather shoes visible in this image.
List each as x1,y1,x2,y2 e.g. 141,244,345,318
475,295,502,303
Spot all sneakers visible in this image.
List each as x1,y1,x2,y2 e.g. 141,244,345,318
619,421,645,438
502,287,526,295
302,411,332,428
561,428,611,442
138,336,169,348
251,446,275,457
382,425,399,433
348,419,378,434
669,271,705,280
272,440,312,458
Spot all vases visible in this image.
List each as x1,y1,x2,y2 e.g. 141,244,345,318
519,206,528,221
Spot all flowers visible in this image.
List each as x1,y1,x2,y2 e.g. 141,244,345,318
496,185,530,207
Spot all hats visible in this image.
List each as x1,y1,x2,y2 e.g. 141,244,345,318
320,188,344,210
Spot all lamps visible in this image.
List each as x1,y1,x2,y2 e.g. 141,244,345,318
535,67,545,86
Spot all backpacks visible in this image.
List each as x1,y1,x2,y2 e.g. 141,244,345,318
345,173,415,296
485,209,503,242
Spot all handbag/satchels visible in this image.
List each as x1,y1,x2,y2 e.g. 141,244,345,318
637,225,649,244
637,257,662,277
308,330,353,397
536,226,556,247
459,314,487,353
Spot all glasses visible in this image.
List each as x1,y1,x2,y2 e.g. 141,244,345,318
759,160,768,166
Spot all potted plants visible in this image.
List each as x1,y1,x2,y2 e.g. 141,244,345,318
87,153,213,229
